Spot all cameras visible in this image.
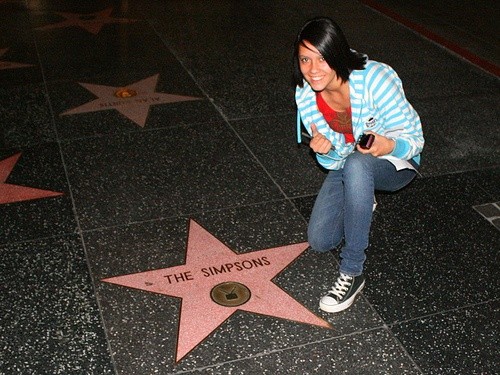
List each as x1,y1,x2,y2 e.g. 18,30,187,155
354,134,375,150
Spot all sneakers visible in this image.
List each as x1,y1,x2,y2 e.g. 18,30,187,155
319,272,365,312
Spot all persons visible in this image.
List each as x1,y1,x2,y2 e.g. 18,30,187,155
291,17,425,313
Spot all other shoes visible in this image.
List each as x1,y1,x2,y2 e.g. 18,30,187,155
373,194,377,211
